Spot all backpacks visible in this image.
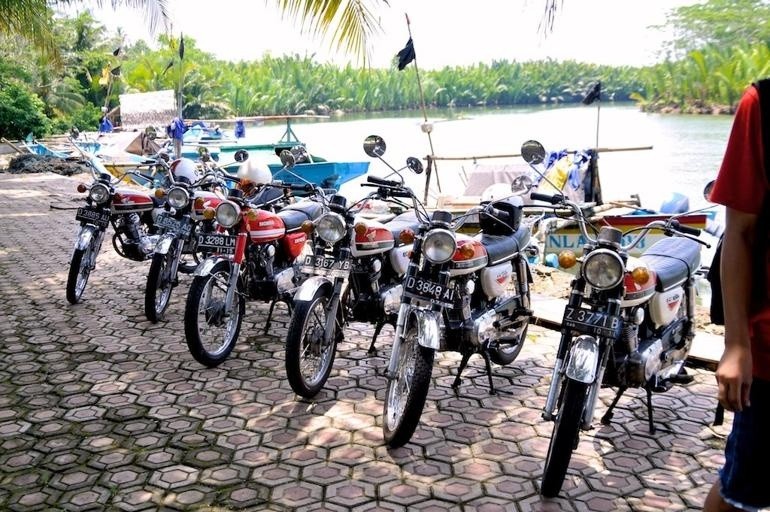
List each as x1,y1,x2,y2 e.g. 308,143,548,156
706,78,770,326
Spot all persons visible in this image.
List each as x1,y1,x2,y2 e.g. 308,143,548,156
703,79,770,512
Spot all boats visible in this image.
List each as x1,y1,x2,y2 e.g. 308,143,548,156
183,124,223,139
